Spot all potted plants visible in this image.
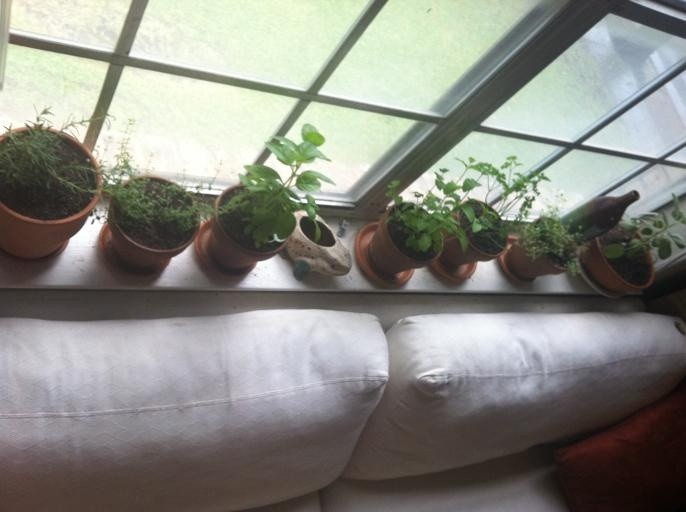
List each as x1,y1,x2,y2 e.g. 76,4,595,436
429,155,548,288
578,186,680,301
498,188,589,291
194,124,337,275
0,103,117,260
99,119,245,270
358,164,483,290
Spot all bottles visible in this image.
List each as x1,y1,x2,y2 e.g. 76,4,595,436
561,190,640,246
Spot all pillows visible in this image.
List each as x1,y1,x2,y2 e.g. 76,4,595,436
0,307,392,512
345,313,684,485
551,383,686,512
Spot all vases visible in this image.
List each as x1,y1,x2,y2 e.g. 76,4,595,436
287,209,352,281
